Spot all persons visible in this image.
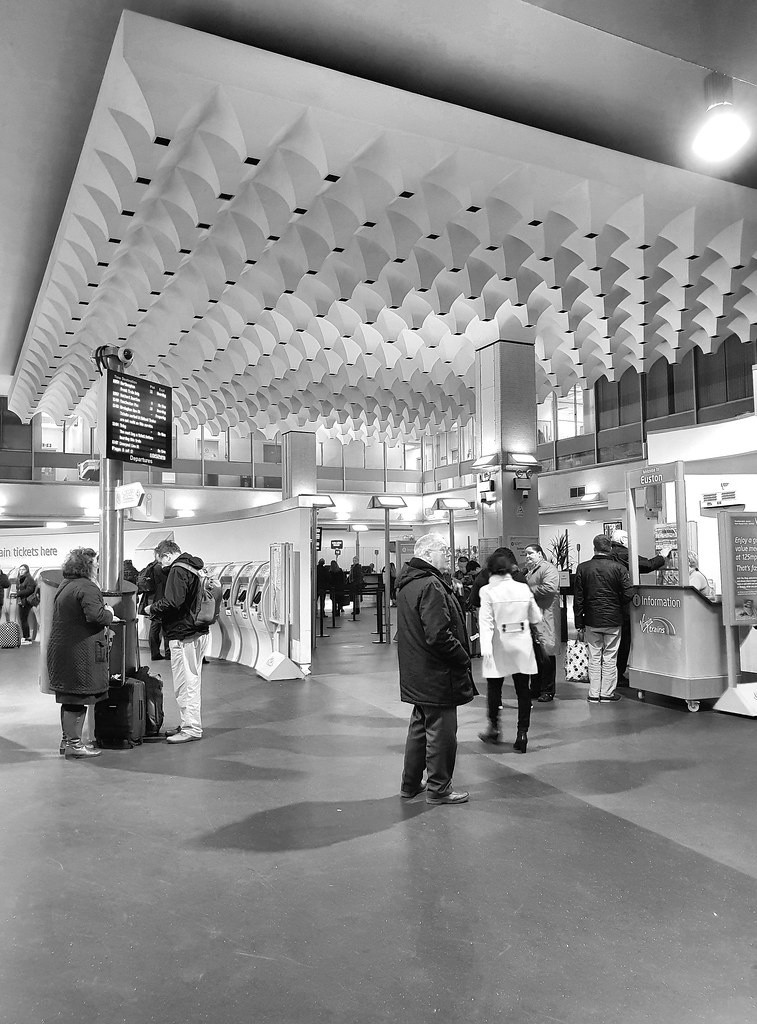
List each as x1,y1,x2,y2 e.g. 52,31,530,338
46,548,115,760
684,550,711,595
316,555,364,618
144,540,209,744
477,553,543,753
123,547,210,664
394,534,481,805
382,562,397,607
609,530,672,687
14,564,35,644
0,569,10,618
446,547,534,709
573,534,634,703
369,563,376,572
524,544,562,702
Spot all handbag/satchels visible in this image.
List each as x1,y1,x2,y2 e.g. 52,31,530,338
531,636,552,668
26,593,39,606
565,629,589,681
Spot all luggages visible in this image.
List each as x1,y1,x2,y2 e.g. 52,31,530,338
463,609,481,658
127,618,164,736
0,593,21,648
94,620,146,749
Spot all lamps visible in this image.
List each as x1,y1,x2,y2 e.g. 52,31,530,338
478,472,494,493
513,468,533,490
692,71,751,164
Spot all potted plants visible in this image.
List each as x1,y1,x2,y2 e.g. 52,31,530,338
545,529,579,642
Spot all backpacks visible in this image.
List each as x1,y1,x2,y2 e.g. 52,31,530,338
172,562,221,626
136,561,157,593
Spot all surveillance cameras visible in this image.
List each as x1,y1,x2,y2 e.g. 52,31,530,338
481,492,486,503
118,348,135,363
523,490,529,499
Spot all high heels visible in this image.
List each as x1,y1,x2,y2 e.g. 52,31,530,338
513,729,527,753
478,727,499,744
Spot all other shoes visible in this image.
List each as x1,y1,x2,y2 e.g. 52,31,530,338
165,726,201,744
400,779,427,798
586,695,600,703
600,693,622,703
152,655,165,660
617,674,629,687
426,790,470,805
538,693,553,702
165,652,171,660
529,689,541,698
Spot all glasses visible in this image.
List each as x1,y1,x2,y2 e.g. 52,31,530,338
428,546,451,555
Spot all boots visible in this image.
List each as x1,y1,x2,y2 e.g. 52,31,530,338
59,706,101,760
